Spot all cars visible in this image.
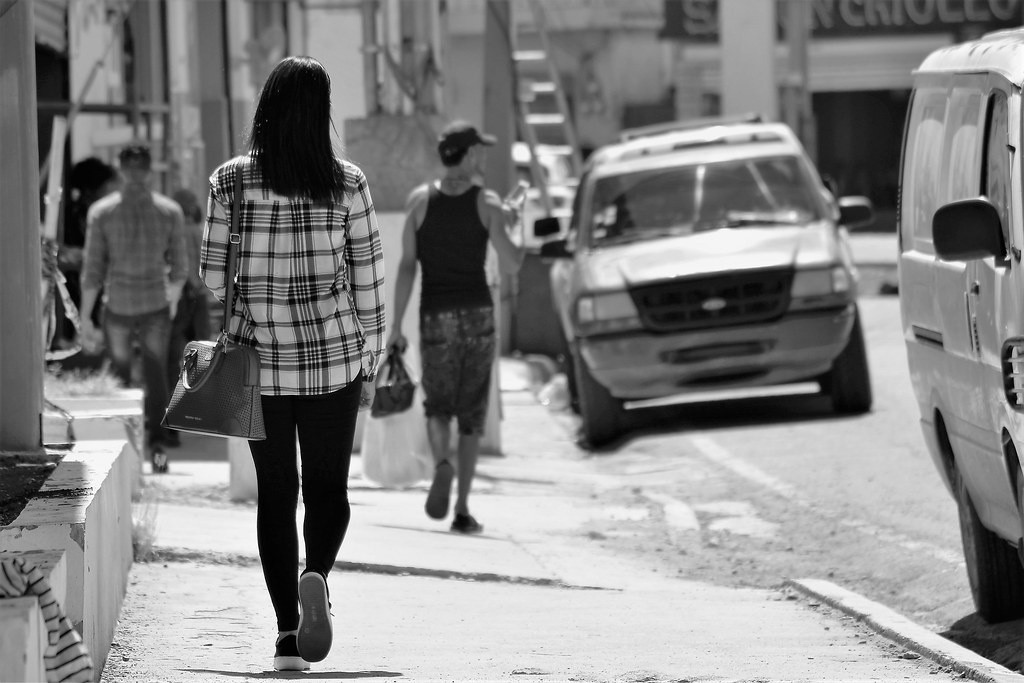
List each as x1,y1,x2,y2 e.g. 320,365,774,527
540,121,872,449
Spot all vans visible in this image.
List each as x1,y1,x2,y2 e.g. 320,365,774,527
897,28,1023,624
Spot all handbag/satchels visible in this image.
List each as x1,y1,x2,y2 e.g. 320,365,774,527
161,340,267,441
371,343,417,417
361,350,435,488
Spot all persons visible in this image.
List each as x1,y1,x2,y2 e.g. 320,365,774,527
383,118,526,536
199,55,388,672
79,145,189,475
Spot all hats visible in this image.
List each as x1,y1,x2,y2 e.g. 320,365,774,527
439,123,498,148
71,157,118,186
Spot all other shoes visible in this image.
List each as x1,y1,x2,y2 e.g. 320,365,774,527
451,513,481,533
426,460,455,519
273,633,311,670
153,447,168,472
296,569,335,662
